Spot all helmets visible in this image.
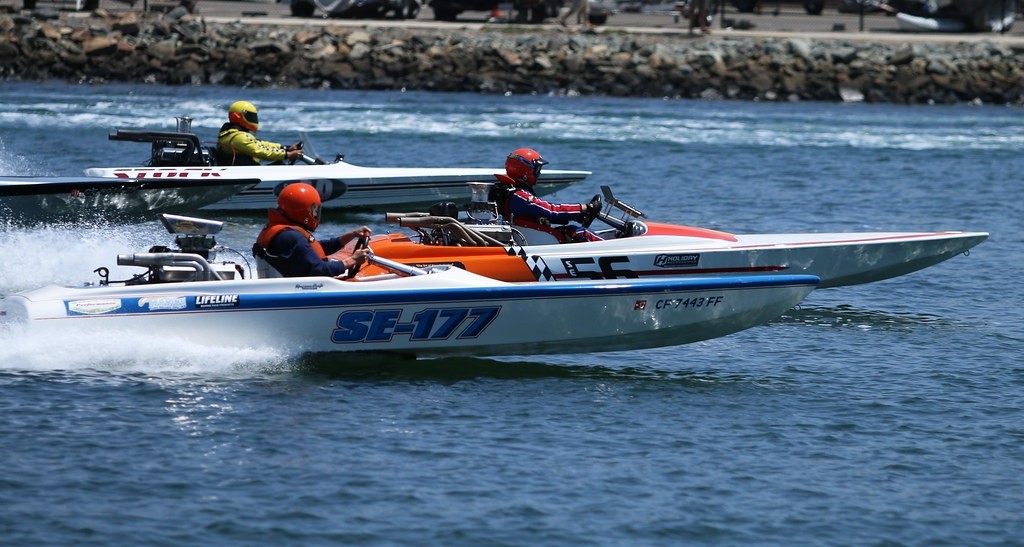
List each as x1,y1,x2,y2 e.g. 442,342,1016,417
506,148,549,186
228,101,259,133
277,182,321,232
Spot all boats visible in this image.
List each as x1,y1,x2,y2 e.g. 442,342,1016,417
327,185,989,282
0,213,820,364
0,117,591,225
895,12,1015,32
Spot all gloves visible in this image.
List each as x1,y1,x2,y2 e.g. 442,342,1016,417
585,200,603,213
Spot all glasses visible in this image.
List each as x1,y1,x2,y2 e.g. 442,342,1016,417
535,165,541,177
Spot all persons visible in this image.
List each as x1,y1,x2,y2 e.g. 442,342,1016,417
487,147,603,244
558,0,590,28
256,182,372,277
217,100,305,166
